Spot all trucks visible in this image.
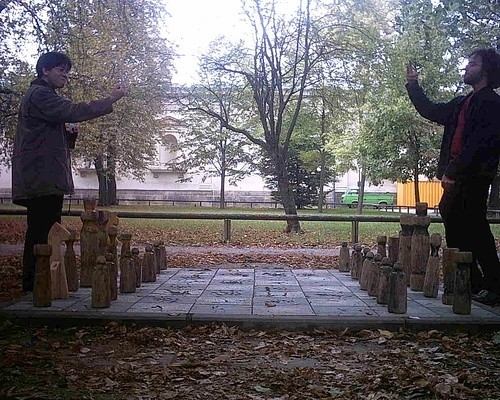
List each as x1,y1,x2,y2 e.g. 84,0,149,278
341,186,395,209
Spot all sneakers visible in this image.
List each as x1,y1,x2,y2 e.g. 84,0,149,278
474,290,500,304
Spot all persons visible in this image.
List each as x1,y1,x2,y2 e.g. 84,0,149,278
405,47,500,307
11,52,126,301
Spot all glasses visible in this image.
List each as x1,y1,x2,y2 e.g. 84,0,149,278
466,64,482,70
53,67,70,75
